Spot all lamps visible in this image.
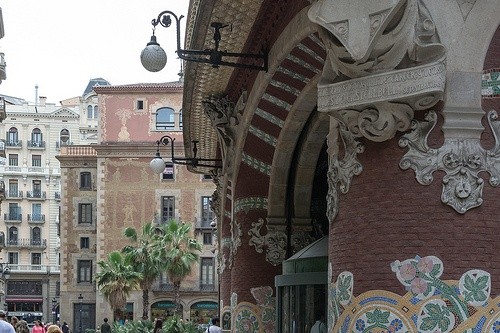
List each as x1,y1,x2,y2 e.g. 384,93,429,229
141,11,269,74
150,136,222,174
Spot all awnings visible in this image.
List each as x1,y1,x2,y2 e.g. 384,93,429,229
152,302,176,308
197,303,218,309
5,298,42,302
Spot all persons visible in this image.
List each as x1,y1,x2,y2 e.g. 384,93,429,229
0,310,73,333
96,318,112,333
180,319,189,324
118,314,129,326
205,317,223,333
141,309,171,333
